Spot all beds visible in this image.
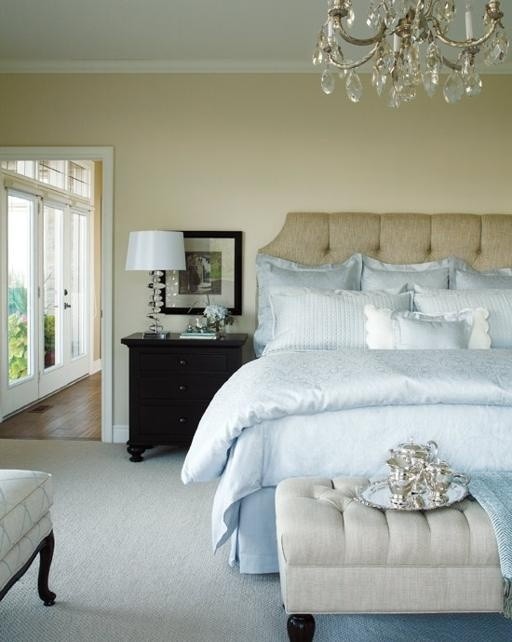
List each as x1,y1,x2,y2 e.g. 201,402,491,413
180,214,512,575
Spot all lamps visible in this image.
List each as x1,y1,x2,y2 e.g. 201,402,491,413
125,230,186,339
311,0,509,109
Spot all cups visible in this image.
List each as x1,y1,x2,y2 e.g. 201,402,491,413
386,462,450,506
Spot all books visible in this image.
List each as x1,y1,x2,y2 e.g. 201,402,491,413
179,330,220,340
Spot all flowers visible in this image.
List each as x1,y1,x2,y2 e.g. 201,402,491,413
188,291,235,332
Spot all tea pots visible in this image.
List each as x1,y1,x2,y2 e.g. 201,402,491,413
388,436,439,496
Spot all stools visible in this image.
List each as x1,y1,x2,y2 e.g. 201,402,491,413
0,469,56,606
273,476,512,642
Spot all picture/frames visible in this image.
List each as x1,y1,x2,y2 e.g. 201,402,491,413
159,230,243,316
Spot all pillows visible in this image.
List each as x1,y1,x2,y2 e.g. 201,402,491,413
411,283,512,348
267,286,412,350
449,255,512,289
363,302,491,351
252,253,362,358
361,255,449,289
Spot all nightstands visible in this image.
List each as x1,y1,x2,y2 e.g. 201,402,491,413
121,332,247,462
